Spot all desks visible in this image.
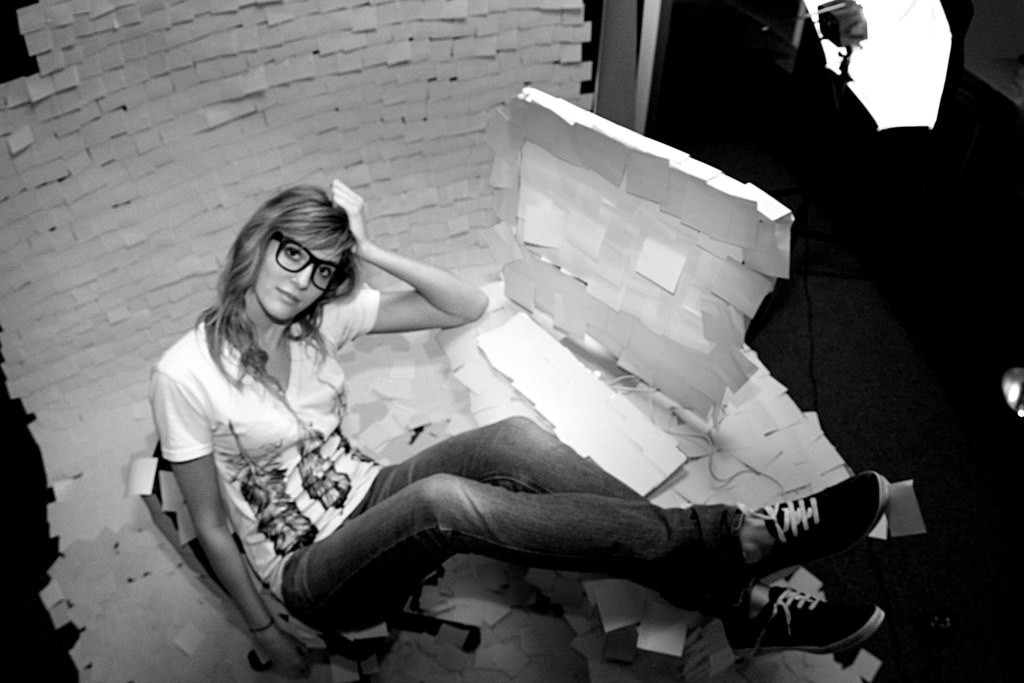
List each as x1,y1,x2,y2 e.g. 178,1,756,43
435,282,855,666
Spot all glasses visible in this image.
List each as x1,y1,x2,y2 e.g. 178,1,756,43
270,231,346,293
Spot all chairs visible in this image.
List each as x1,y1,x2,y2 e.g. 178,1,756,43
141,440,482,683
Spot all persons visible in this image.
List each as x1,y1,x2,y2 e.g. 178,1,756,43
149,177,891,679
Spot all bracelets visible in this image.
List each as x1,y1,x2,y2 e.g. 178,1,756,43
250,618,275,632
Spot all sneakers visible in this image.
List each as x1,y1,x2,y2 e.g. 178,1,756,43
722,583,884,654
736,471,891,584
